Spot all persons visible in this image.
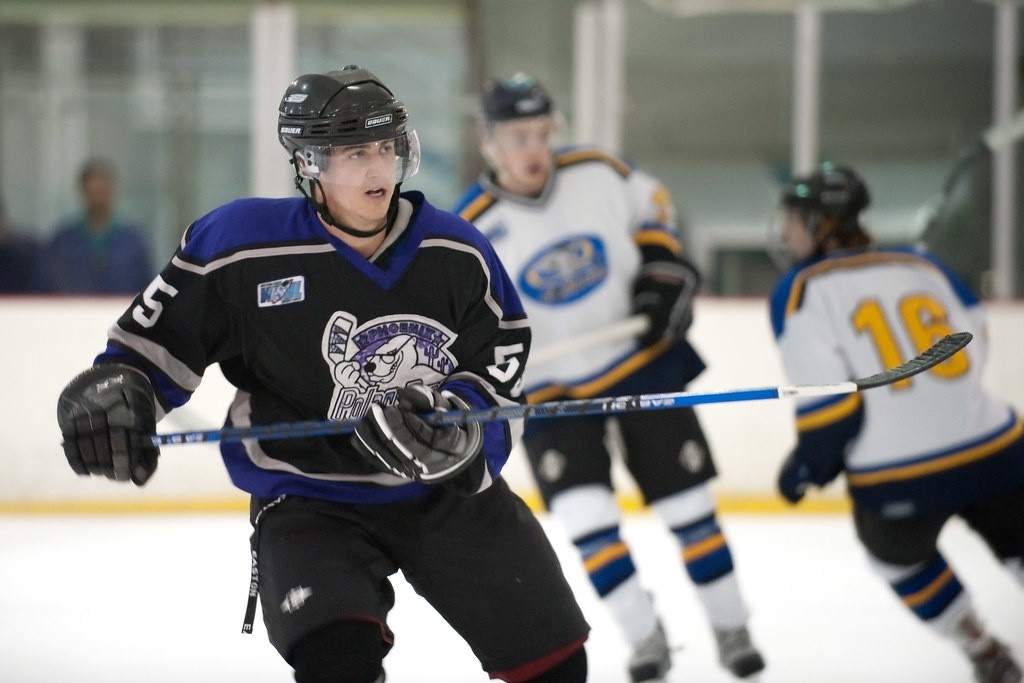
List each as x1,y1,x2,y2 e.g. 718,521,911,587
55,65,589,683
449,76,766,683
770,159,1024,683
0,157,151,298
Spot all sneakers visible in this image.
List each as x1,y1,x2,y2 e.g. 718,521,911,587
627,620,672,683
714,625,766,682
969,638,1022,682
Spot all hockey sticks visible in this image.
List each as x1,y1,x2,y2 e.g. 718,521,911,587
151,333,973,446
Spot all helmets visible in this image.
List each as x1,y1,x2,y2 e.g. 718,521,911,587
779,159,869,211
479,71,551,121
277,65,408,174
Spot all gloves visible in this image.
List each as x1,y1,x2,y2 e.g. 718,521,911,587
57,363,161,486
778,445,820,503
631,266,697,346
350,384,484,485
